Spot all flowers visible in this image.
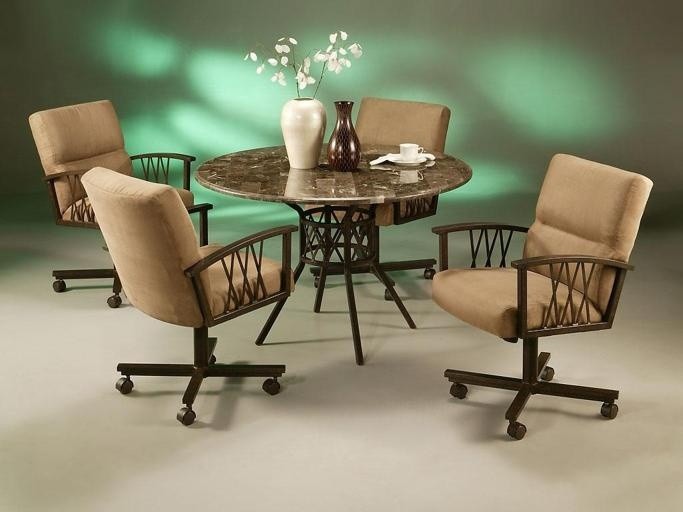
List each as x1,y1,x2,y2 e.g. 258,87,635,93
243,29,363,100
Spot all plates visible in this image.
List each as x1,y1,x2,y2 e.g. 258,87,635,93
388,154,427,166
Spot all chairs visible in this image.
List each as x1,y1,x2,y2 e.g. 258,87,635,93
28,99,212,308
76,165,301,424
310,95,450,298
429,153,654,438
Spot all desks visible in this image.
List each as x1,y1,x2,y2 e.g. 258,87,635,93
195,141,472,364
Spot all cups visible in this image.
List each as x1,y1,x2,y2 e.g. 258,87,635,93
399,143,423,162
399,170,423,183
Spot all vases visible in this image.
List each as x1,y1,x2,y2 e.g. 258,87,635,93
327,101,360,173
278,98,325,166
